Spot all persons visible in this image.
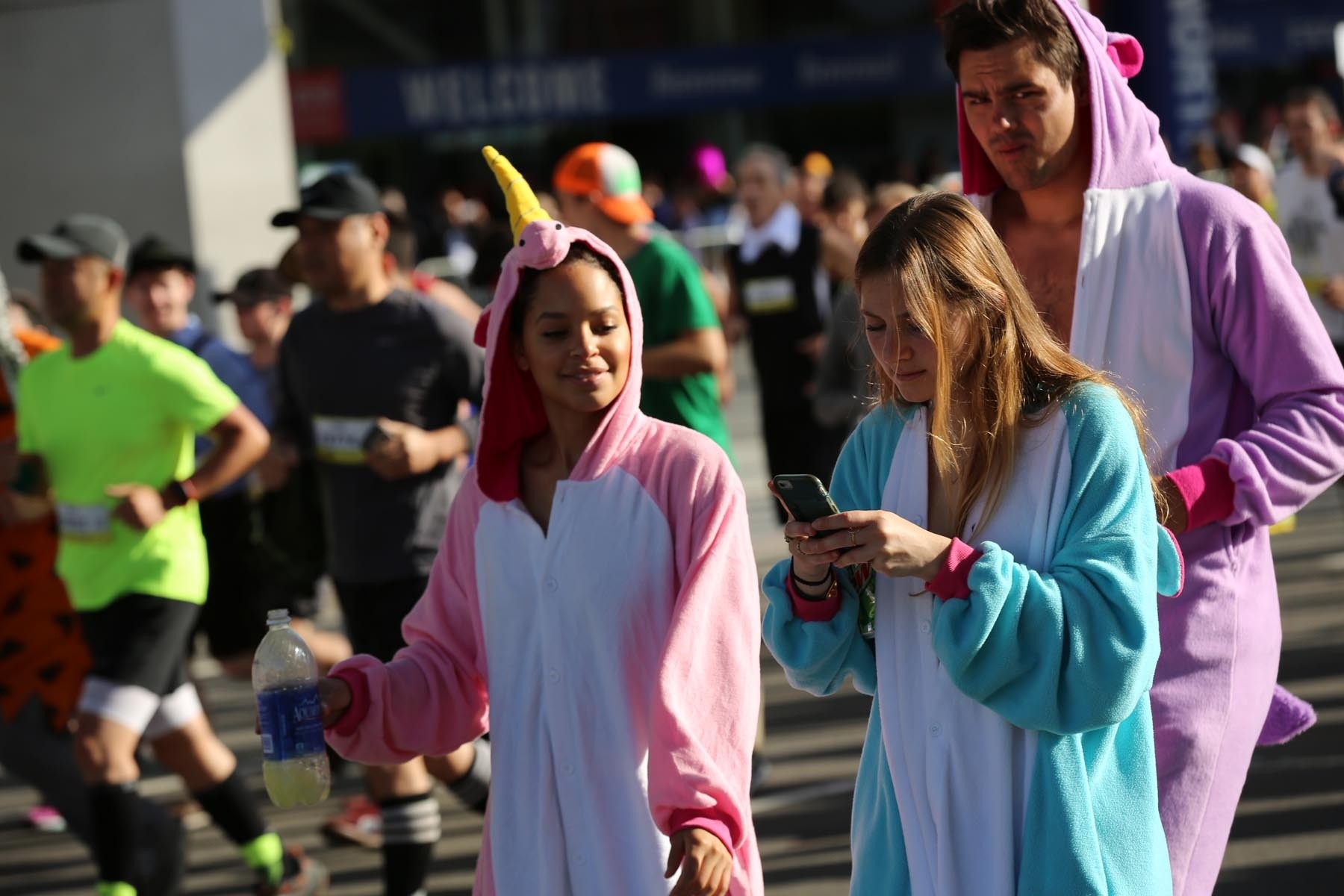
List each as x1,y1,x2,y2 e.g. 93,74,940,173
1,2,1344,896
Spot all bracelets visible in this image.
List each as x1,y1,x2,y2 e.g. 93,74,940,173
169,479,190,506
795,580,839,600
790,555,832,585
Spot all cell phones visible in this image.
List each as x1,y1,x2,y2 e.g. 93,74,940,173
773,474,856,555
360,425,388,454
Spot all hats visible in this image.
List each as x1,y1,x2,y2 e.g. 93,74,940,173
15,212,129,267
552,141,654,226
271,171,383,227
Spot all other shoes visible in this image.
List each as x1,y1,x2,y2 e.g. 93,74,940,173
22,804,68,832
319,786,386,851
168,799,216,832
253,843,330,896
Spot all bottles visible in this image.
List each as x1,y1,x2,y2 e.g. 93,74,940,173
252,609,331,809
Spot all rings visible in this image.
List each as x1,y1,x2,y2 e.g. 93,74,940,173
796,539,806,555
784,522,794,542
848,527,860,546
